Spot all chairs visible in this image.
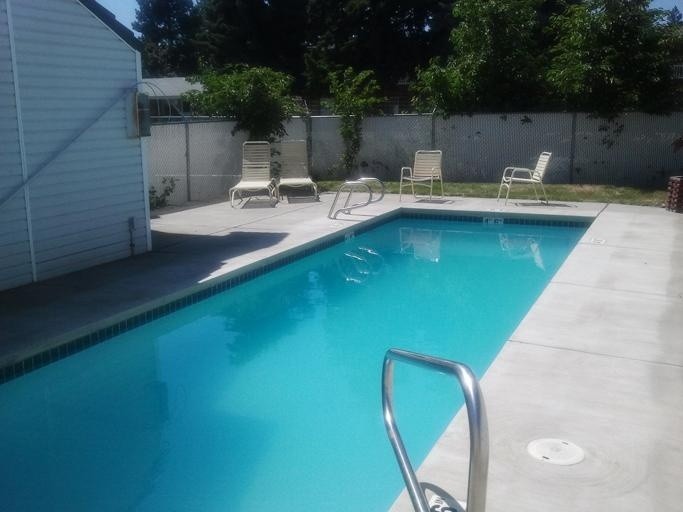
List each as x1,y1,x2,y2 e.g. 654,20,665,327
398,149,445,204
494,233,546,275
228,139,318,209
496,151,553,206
396,222,444,267
